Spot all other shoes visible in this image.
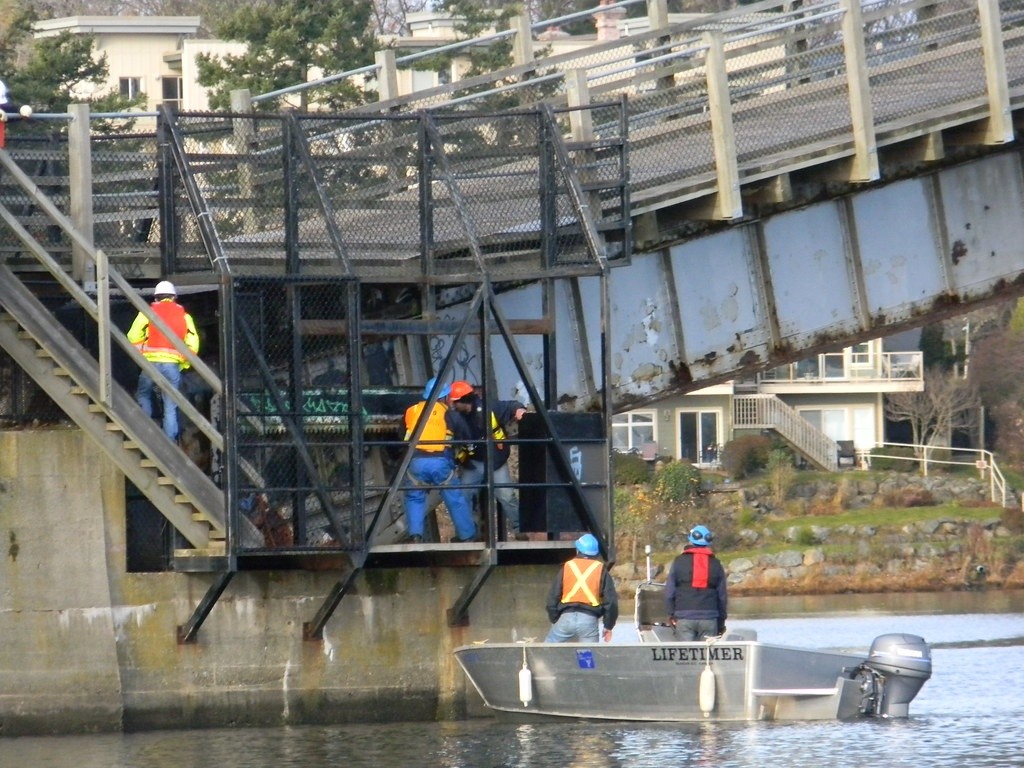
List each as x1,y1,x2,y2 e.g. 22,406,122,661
515,533,529,541
473,536,489,542
463,536,476,543
411,534,421,544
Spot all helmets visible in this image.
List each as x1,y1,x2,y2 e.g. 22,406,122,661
422,376,451,401
575,534,599,556
0,81,8,104
688,525,713,546
154,280,177,295
447,380,474,401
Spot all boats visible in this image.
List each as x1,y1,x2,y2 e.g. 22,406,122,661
452,543,933,726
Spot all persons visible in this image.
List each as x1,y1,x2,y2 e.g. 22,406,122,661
447,382,529,542
542,533,618,643
663,525,727,641
397,377,474,546
128,280,199,442
